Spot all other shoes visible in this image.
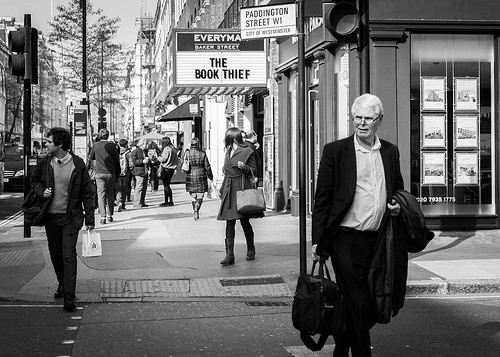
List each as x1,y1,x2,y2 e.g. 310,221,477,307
63,293,77,312
117,202,126,212
99,219,106,225
54,283,63,298
107,217,113,222
159,202,174,207
127,200,131,202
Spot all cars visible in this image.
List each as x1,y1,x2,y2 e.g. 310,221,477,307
0,145,25,192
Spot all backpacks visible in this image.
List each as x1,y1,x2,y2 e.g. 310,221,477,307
119,150,130,176
21,155,51,227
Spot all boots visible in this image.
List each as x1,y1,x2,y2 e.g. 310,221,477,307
192,198,204,220
244,232,256,261
220,239,235,265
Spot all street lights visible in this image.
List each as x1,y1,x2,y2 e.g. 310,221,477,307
51,109,58,128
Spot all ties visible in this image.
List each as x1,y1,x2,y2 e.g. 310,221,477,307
58,160,62,164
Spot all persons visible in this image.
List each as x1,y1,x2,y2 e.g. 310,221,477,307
219,127,257,265
181,138,214,220
87,135,178,212
30,128,95,312
312,94,405,357
244,131,261,191
89,129,121,224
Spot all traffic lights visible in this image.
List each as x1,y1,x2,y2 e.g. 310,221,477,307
8,27,39,84
322,0,360,42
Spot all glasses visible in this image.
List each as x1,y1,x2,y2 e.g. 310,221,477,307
353,114,380,125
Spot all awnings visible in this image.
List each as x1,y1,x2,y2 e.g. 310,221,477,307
156,96,201,120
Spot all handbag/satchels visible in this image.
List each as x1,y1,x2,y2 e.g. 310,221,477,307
207,181,221,200
236,165,266,213
181,152,189,171
292,260,337,351
82,228,103,257
